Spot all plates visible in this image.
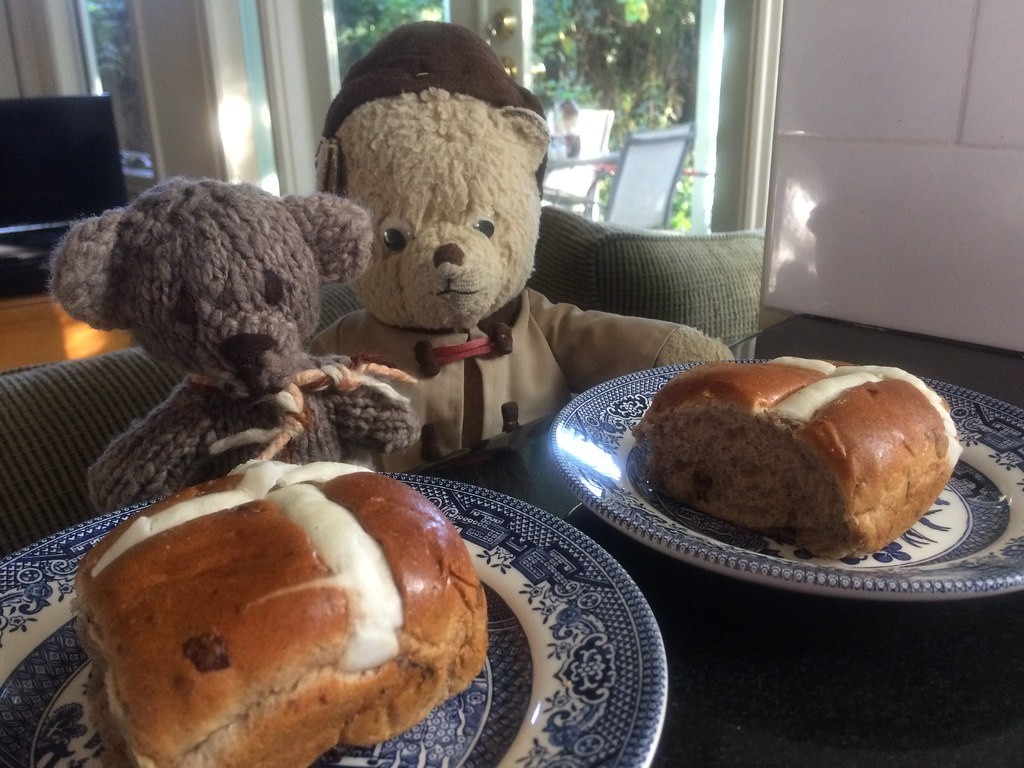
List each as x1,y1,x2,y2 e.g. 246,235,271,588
550,355,1024,599
0,472,669,768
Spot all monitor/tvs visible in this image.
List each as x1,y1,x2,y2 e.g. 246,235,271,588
0,93,127,237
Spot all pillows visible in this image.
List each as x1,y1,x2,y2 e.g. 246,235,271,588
526,206,769,363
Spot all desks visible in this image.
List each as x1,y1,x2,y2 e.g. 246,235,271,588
0,315,1024,768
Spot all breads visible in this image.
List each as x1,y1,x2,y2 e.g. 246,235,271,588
634,356,962,559
70,459,487,768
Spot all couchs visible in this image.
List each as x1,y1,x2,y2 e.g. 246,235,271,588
0,202,768,564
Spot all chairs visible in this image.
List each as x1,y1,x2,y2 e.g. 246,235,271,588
539,109,615,221
0,94,131,303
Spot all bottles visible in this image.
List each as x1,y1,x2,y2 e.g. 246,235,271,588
561,93,581,156
547,103,567,161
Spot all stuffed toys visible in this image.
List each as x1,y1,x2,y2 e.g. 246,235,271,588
311,20,734,472
49,176,419,514
